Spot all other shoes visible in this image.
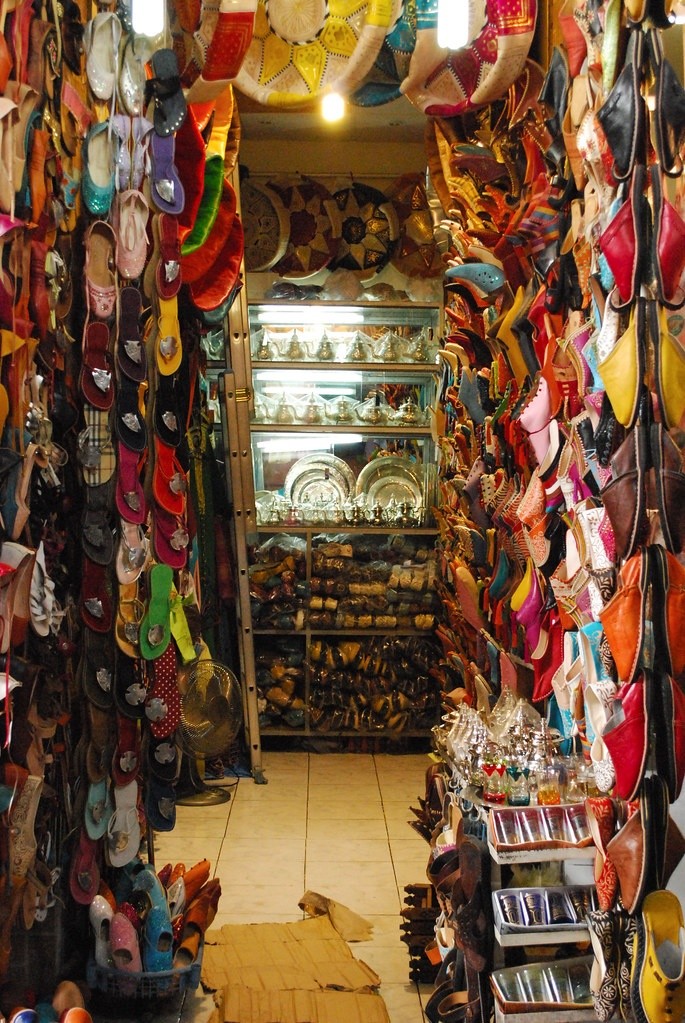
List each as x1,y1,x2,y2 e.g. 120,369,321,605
250,529,453,732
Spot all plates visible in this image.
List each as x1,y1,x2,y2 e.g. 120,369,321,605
368,477,422,519
356,457,425,519
284,453,356,513
298,477,346,513
284,464,348,517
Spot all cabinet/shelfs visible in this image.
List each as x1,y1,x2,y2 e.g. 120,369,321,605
205,299,445,753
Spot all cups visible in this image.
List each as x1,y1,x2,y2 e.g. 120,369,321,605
496,812,516,844
521,967,544,1001
545,964,571,1002
525,893,546,925
549,892,568,924
546,807,566,840
498,972,522,1001
571,893,592,921
519,809,541,842
483,759,604,804
503,894,520,924
570,965,592,1003
569,806,592,840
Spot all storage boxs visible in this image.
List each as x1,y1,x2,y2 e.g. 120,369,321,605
488,955,599,1023
492,887,596,948
487,800,597,864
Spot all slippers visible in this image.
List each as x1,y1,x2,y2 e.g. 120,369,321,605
414,0,685,1023
0,1,223,1023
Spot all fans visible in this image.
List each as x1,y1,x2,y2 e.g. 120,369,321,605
173,659,244,807
